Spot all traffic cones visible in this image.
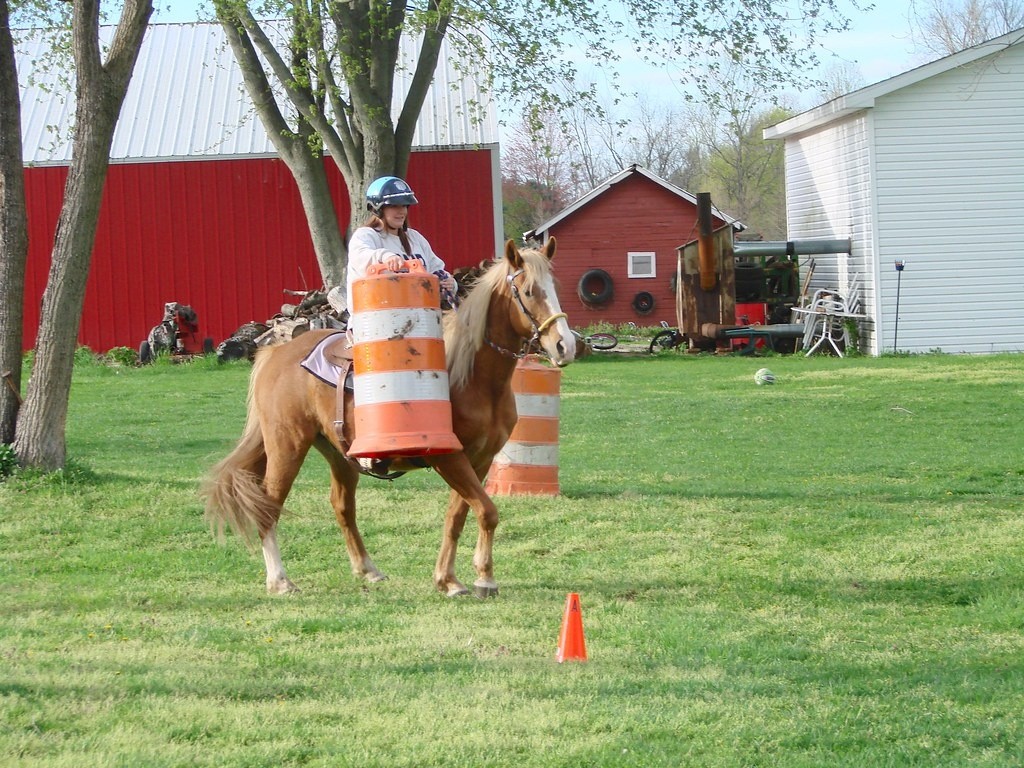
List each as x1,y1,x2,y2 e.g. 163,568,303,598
554,592,589,662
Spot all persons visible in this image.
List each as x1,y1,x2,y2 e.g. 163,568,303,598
344,177,462,335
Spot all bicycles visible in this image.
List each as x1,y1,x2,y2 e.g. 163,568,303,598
571,329,618,350
648,321,685,354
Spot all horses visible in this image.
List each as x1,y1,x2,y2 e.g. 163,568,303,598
193,236,577,601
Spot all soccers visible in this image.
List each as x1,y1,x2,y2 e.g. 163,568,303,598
753,368,775,387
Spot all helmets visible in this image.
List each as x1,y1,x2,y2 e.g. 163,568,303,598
366,176,419,211
